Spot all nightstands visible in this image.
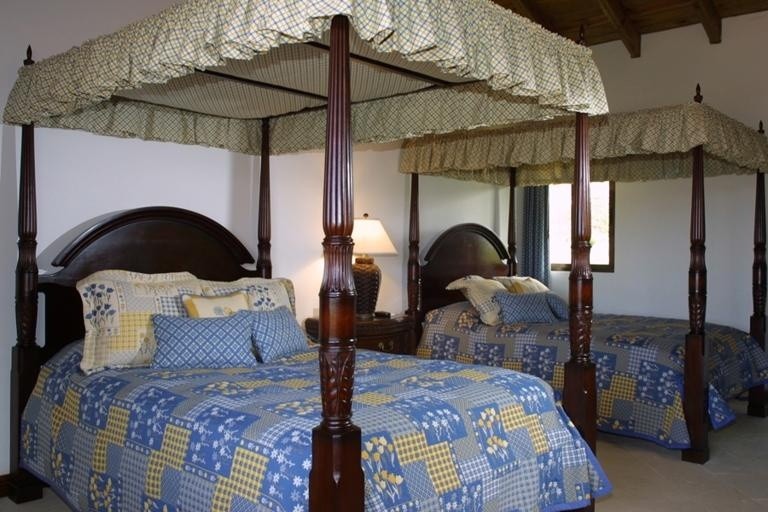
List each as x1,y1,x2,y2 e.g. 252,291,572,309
305,314,415,354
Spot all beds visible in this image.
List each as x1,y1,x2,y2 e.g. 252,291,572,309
9,0,609,512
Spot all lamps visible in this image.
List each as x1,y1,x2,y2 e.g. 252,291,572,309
350,214,396,318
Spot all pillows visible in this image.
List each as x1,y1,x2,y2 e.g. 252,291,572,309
496,290,558,325
492,275,550,293
445,274,514,327
150,313,258,370
199,277,297,315
545,290,570,322
75,270,206,374
238,305,311,363
512,278,540,293
181,289,250,318
